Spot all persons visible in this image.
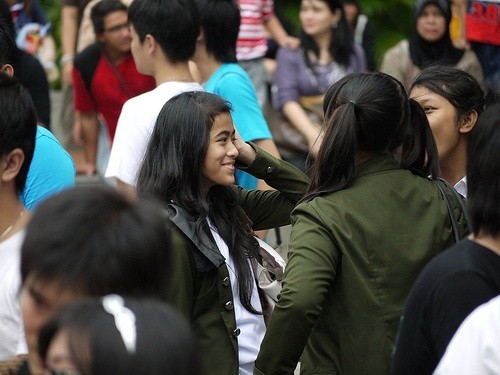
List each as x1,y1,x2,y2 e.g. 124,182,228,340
273,0,368,183
104,0,205,201
432,296,500,375
0,74,50,375
7,0,136,158
0,0,76,214
20,181,169,374
73,0,159,180
179,0,282,246
40,300,196,375
235,0,300,110
451,0,500,101
406,64,479,204
341,0,374,71
382,0,482,94
252,71,471,375
136,93,312,375
390,103,500,375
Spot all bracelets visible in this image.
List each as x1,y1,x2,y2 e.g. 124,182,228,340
59,54,73,67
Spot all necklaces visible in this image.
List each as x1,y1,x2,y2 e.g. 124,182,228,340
0,207,26,241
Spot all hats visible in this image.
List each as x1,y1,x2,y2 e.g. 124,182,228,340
414,0,449,20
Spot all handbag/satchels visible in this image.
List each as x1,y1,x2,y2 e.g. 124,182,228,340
250,234,287,308
262,83,327,153
